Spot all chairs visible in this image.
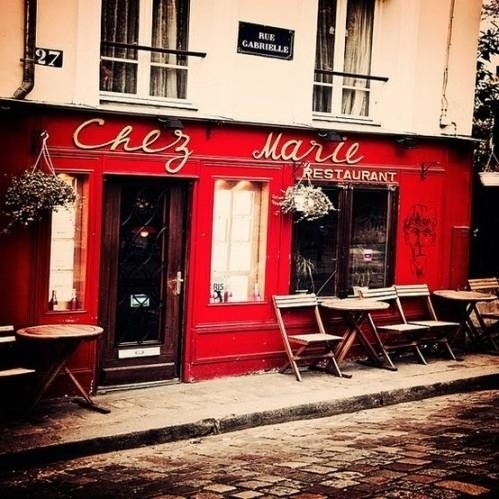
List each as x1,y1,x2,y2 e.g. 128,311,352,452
1,327,37,415
273,293,342,382
394,284,461,366
467,279,499,356
358,286,428,369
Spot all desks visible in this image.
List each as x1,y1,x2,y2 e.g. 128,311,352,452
18,323,110,415
431,289,498,359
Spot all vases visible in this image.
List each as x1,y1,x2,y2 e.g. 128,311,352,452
479,172,499,186
293,196,313,210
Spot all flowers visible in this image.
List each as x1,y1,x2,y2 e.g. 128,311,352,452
2,172,83,232
271,183,338,229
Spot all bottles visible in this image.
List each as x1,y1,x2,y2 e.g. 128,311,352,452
70,289,79,311
49,290,57,312
